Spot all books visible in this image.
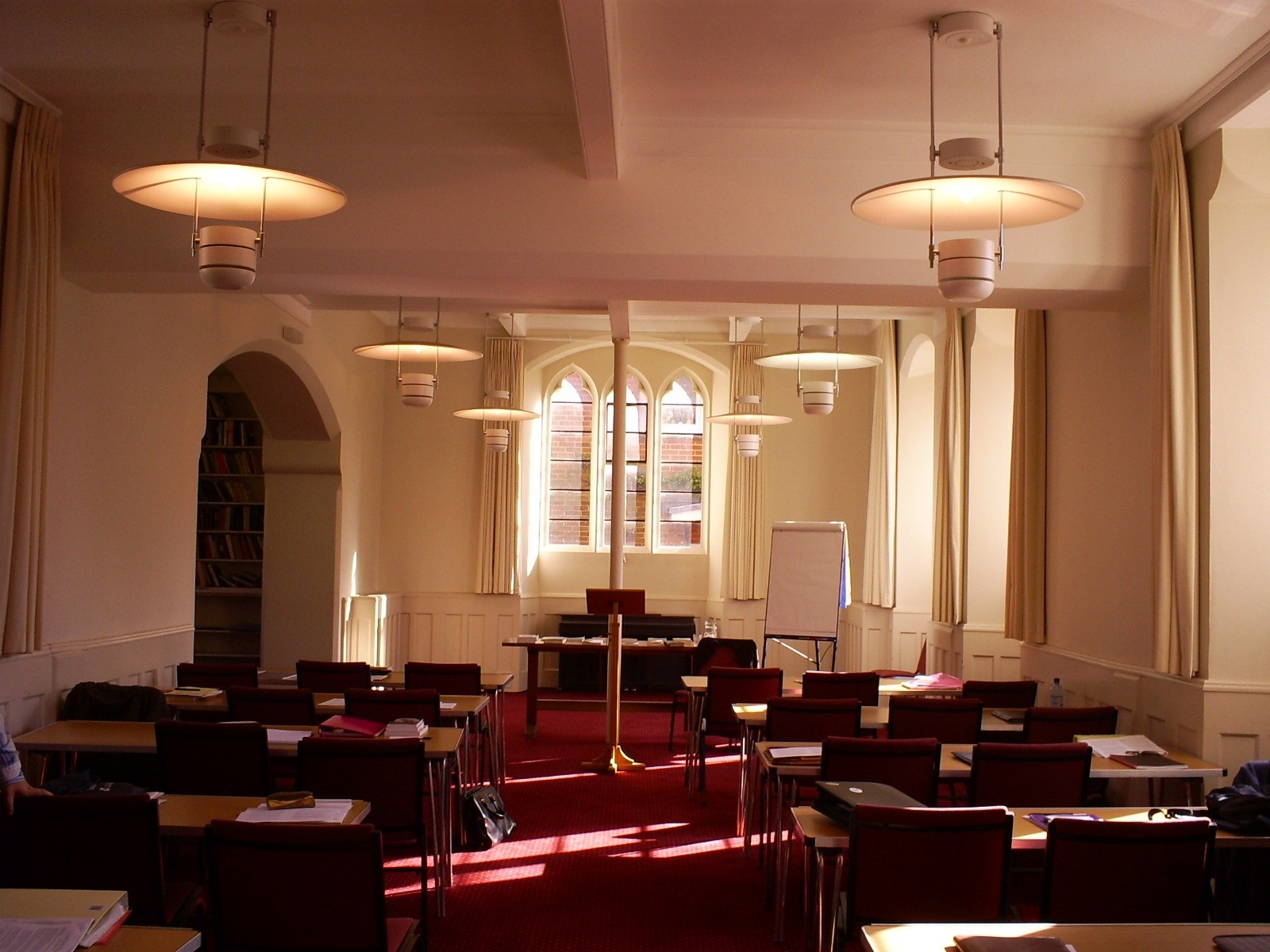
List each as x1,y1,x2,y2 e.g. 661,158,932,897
385,718,428,737
196,393,264,588
544,636,567,644
582,639,603,645
517,634,536,643
1111,753,1188,769
648,638,691,646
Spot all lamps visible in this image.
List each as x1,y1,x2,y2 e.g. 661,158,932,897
705,304,883,456
112,0,347,289
353,297,539,452
852,13,1083,303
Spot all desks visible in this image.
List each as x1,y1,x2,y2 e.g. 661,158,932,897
779,805,1270,952
502,638,698,738
860,923,1270,952
732,703,1028,854
161,690,490,850
74,925,201,952
158,794,372,837
755,742,1229,944
258,667,514,794
13,721,465,919
680,678,962,802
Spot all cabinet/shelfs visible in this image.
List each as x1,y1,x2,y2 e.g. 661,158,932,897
196,392,265,598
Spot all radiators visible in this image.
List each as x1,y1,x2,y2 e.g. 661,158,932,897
843,602,886,672
349,592,403,671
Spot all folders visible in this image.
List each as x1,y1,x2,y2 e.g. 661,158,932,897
0,888,129,948
318,715,387,738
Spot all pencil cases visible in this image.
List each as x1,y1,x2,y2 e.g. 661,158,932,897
266,792,315,810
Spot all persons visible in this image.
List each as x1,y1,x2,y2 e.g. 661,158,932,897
0,713,53,816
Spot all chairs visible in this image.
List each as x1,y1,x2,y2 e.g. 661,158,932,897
668,637,1270,952
0,660,494,952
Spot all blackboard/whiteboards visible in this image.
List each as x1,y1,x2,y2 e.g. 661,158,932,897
764,528,845,641
